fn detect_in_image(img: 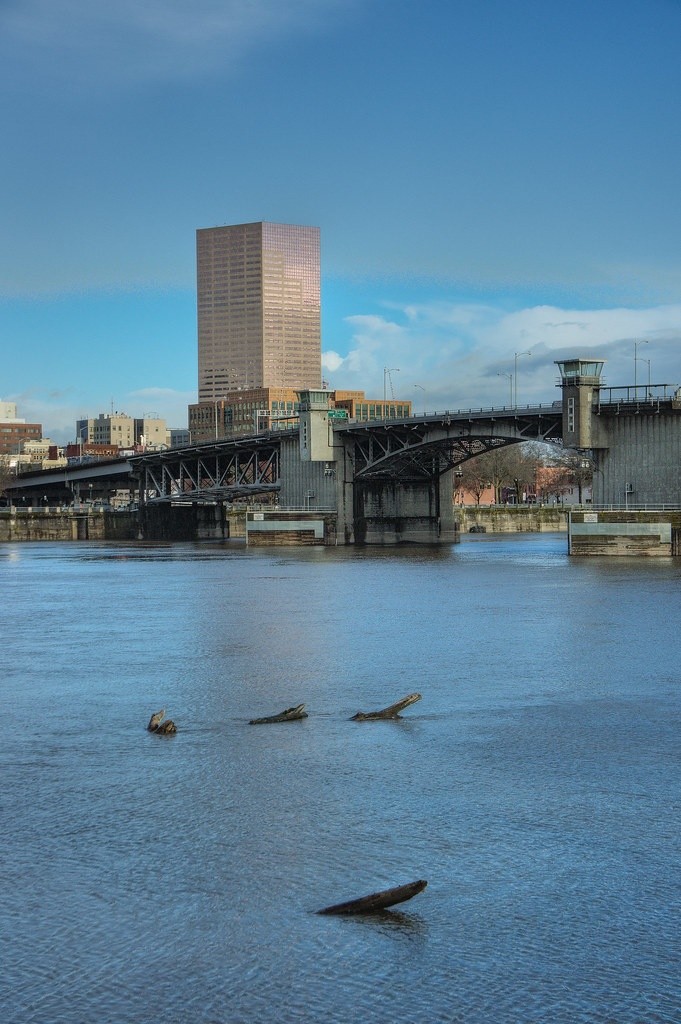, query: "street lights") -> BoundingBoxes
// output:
[415,383,426,414]
[514,350,531,410]
[383,367,400,419]
[634,358,650,397]
[635,340,649,400]
[456,465,463,504]
[89,483,93,499]
[18,437,31,472]
[215,397,227,439]
[80,426,94,462]
[497,371,513,409]
